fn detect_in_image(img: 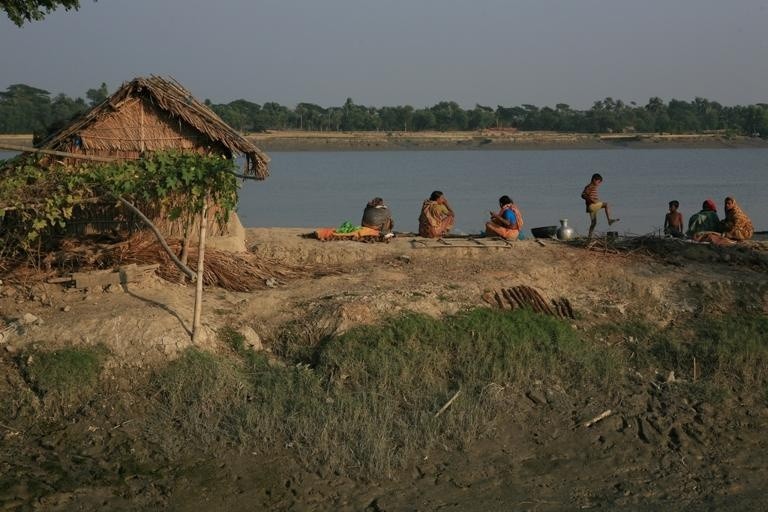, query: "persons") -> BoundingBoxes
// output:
[663,200,684,237]
[581,173,621,239]
[719,196,755,241]
[417,191,455,239]
[485,195,523,241]
[361,197,395,238]
[685,199,721,235]
